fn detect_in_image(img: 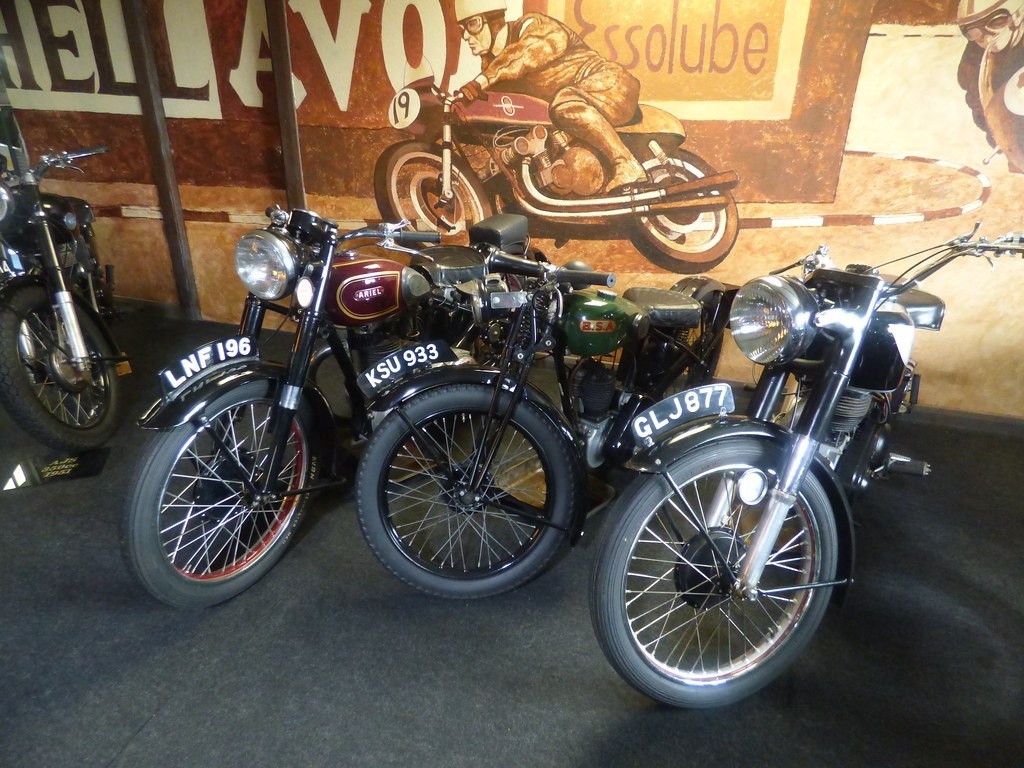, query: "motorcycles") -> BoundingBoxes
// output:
[114,201,554,612]
[591,212,1024,712]
[345,228,748,604]
[0,144,129,451]
[368,56,745,279]
